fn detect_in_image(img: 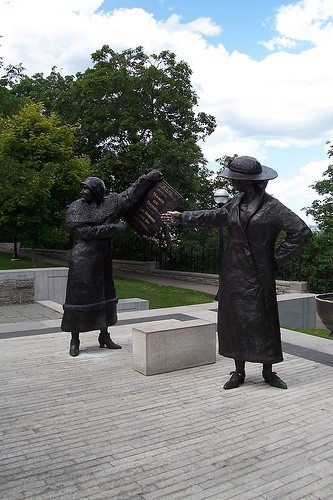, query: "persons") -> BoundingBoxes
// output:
[160,156,311,389]
[60,168,163,357]
